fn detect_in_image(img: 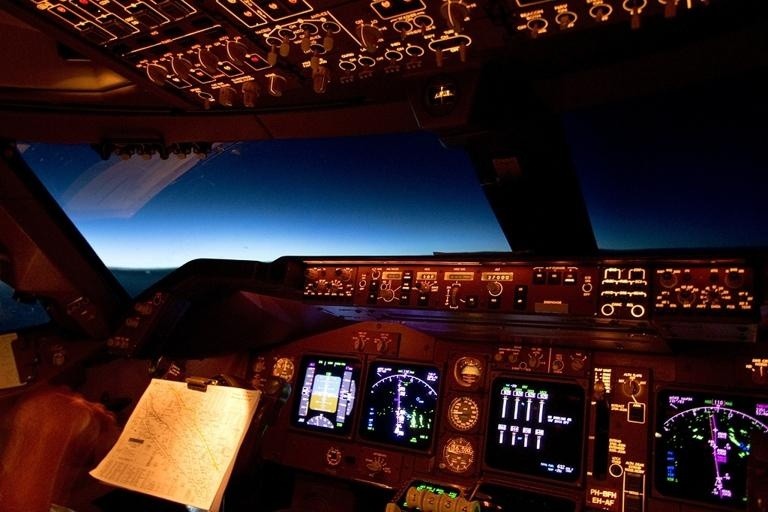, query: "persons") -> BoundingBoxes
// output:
[1,368,119,510]
